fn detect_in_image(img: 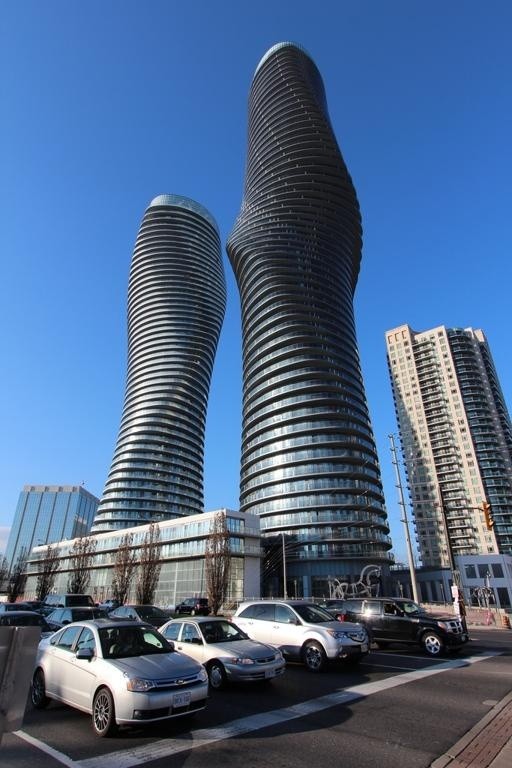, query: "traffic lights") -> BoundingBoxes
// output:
[481,500,496,531]
